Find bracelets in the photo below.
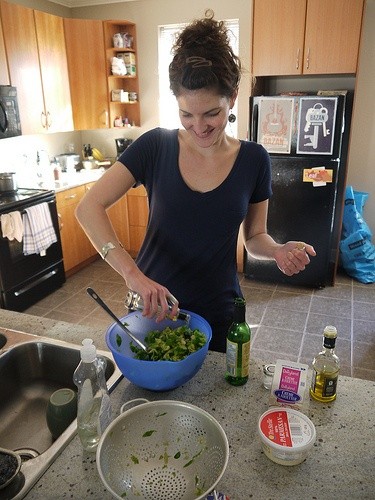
[100,241,124,260]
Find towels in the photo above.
[23,201,58,257]
[1,211,23,243]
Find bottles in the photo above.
[112,33,124,48]
[54,163,60,184]
[122,292,191,322]
[72,338,113,453]
[224,297,252,386]
[309,324,341,403]
[46,388,78,439]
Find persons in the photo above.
[74,19,316,353]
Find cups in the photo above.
[262,363,275,390]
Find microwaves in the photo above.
[0,86,22,140]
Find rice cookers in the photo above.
[54,153,81,174]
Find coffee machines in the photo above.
[115,138,132,160]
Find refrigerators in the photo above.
[243,92,354,290]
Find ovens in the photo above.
[0,200,66,312]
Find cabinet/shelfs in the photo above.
[251,0,365,77]
[0,0,143,135]
[57,178,151,280]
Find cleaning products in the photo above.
[73,338,113,454]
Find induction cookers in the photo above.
[0,188,56,216]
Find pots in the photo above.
[81,160,111,169]
[0,172,17,192]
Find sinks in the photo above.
[0,333,7,351]
[0,341,123,500]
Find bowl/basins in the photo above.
[104,308,212,392]
[258,407,316,467]
